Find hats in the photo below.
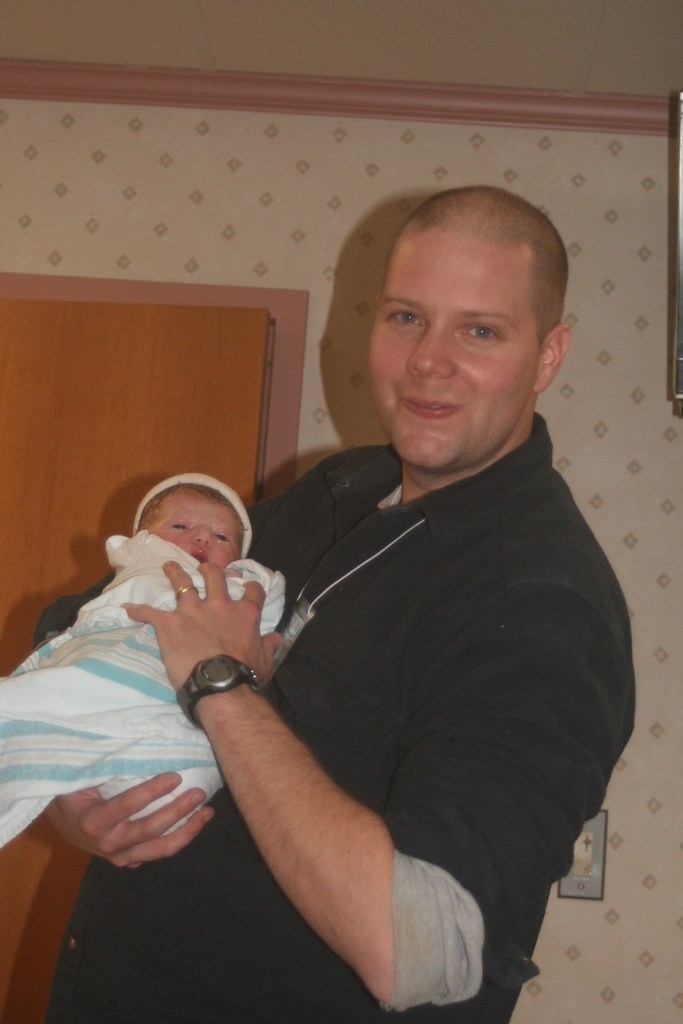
[132,472,252,559]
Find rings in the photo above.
[176,585,198,599]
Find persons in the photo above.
[0,473,285,850]
[34,185,636,1024]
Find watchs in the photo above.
[176,654,260,729]
